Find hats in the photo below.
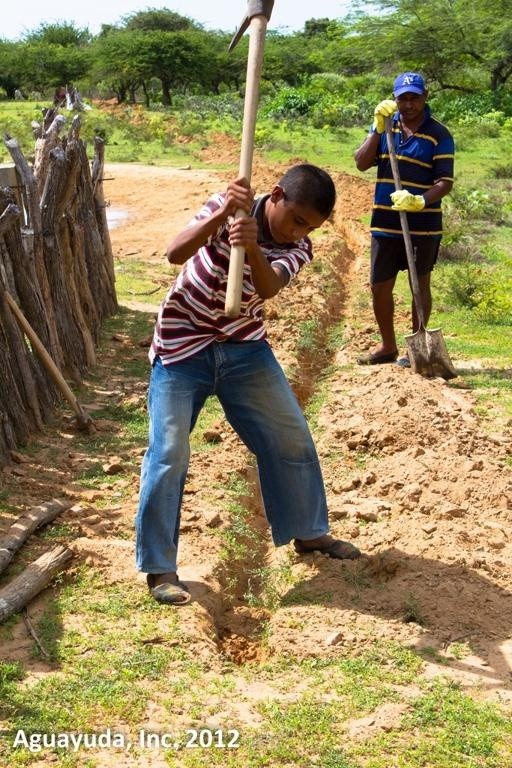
[393,72,425,97]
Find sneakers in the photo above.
[356,348,412,368]
[146,573,192,605]
[293,535,362,560]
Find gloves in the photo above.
[389,189,426,213]
[373,100,398,134]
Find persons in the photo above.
[136,164,361,606]
[354,72,455,368]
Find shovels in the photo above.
[384,114,458,379]
